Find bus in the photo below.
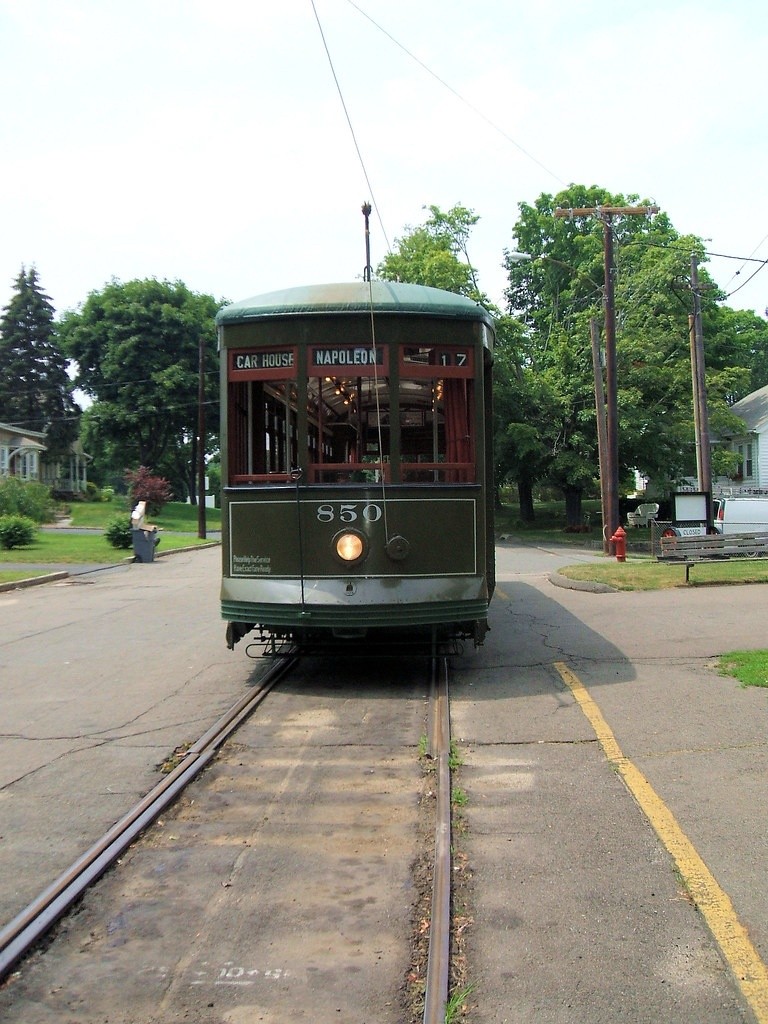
[215,201,497,665]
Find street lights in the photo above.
[508,251,619,558]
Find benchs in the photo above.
[656,531,768,583]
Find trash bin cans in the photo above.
[129,528,157,562]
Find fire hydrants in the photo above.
[609,525,628,564]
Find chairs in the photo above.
[627,503,660,528]
